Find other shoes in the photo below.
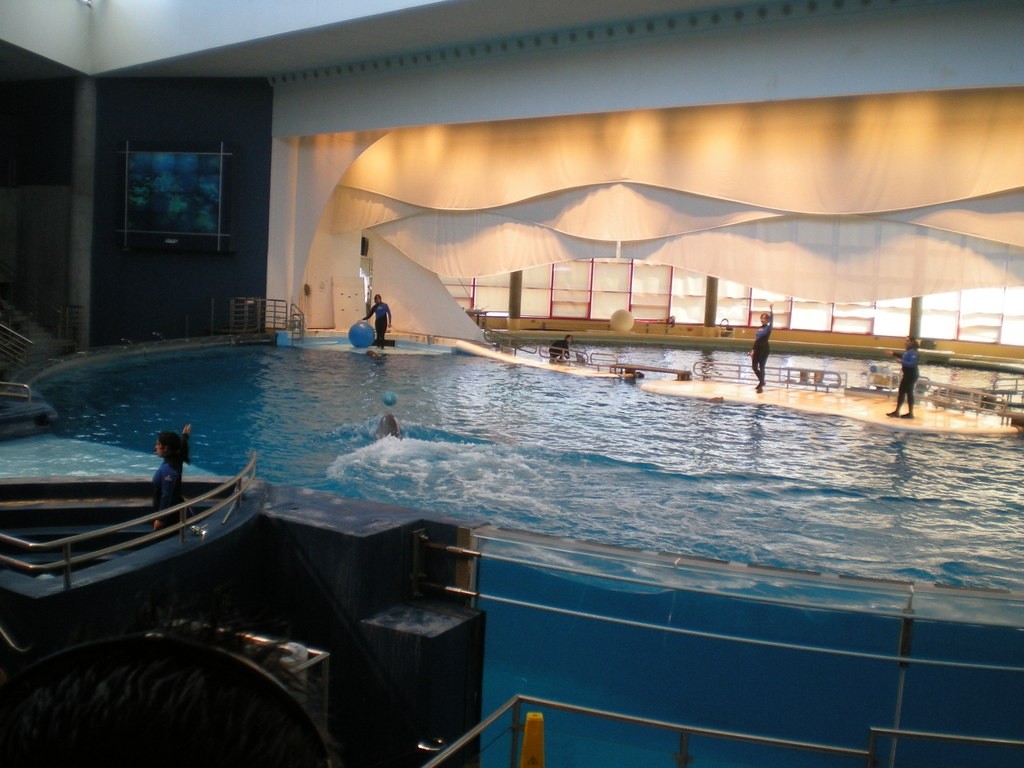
[901,413,913,418]
[887,412,899,417]
[756,383,765,389]
[757,388,762,393]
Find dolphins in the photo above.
[373,411,402,440]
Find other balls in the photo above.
[383,390,399,407]
[349,322,376,348]
[610,310,635,332]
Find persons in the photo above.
[153,424,192,538]
[358,294,391,349]
[549,335,573,363]
[883,336,920,418]
[750,303,774,393]
